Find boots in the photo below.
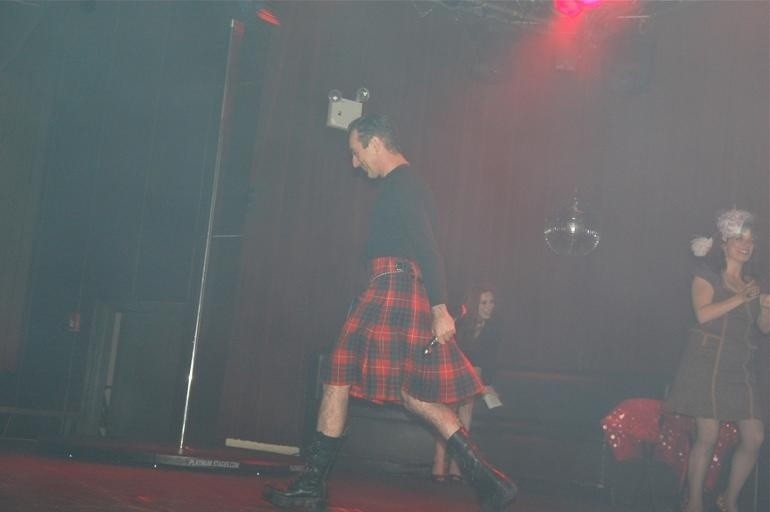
[446,425,518,511]
[262,430,344,512]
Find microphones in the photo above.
[423,303,469,356]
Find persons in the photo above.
[258,110,519,512]
[429,285,502,485]
[675,206,770,512]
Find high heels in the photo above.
[431,459,462,484]
[682,493,727,511]
